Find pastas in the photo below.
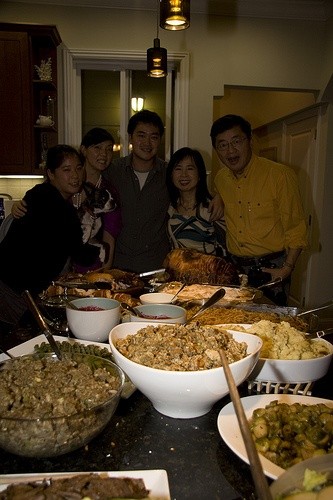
[182,305,310,333]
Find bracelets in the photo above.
[283,261,296,271]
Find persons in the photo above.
[0,109,308,332]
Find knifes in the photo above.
[25,290,63,361]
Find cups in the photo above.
[39,115,54,127]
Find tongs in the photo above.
[54,267,113,290]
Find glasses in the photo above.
[216,137,247,151]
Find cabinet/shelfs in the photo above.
[0,22,62,175]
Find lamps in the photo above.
[146,6,168,77]
[160,0,190,32]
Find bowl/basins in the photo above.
[140,293,178,305]
[215,324,333,383]
[0,352,125,460]
[130,304,187,324]
[109,322,264,418]
[269,454,333,500]
[66,298,121,342]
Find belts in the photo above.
[234,252,284,266]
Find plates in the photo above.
[114,279,145,293]
[155,284,321,334]
[0,470,170,500]
[0,334,136,397]
[217,394,333,480]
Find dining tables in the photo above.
[0,271,333,500]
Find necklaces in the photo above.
[179,204,195,211]
